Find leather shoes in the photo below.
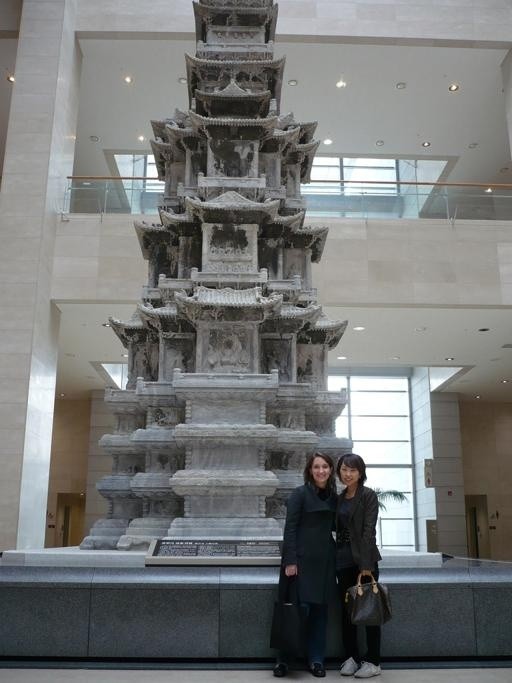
[273,660,290,677]
[308,663,326,677]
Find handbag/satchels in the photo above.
[269,598,310,659]
[344,571,392,626]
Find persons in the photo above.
[335,454,382,678]
[273,451,339,677]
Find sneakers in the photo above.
[354,661,381,678]
[338,658,359,675]
